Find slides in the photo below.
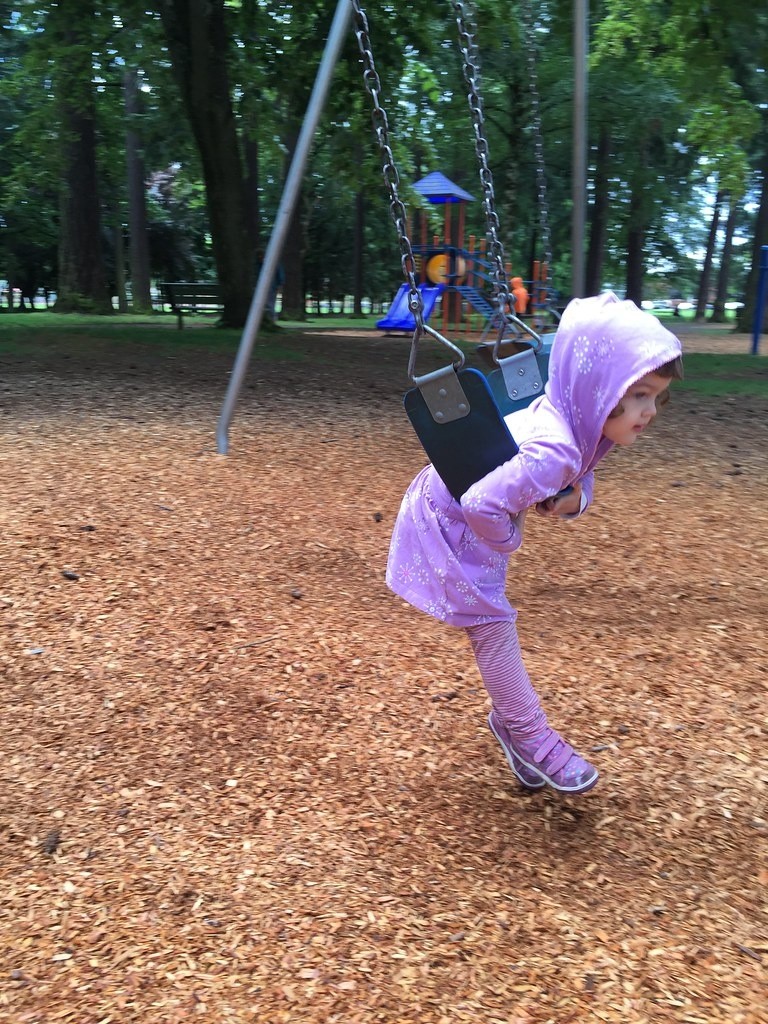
[377,281,444,334]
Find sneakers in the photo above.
[487,710,546,789]
[510,728,599,794]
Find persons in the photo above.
[508,275,529,313]
[385,290,685,792]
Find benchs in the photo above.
[157,283,225,329]
[515,314,545,336]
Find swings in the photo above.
[351,0,573,506]
